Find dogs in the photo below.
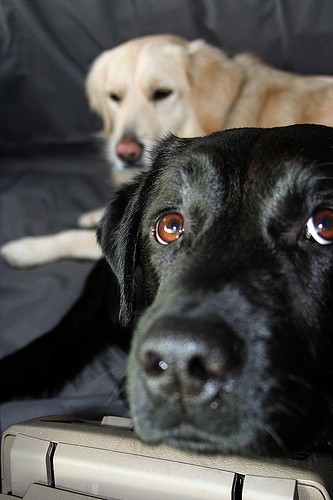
[0,30,332,269]
[0,122,331,461]
